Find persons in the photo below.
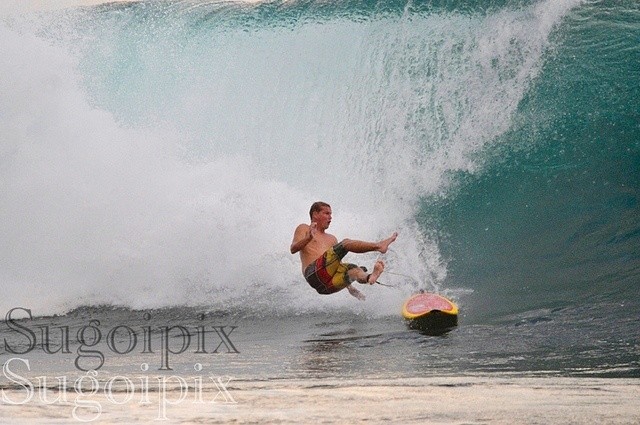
[290,202,399,301]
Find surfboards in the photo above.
[403,294,457,320]
